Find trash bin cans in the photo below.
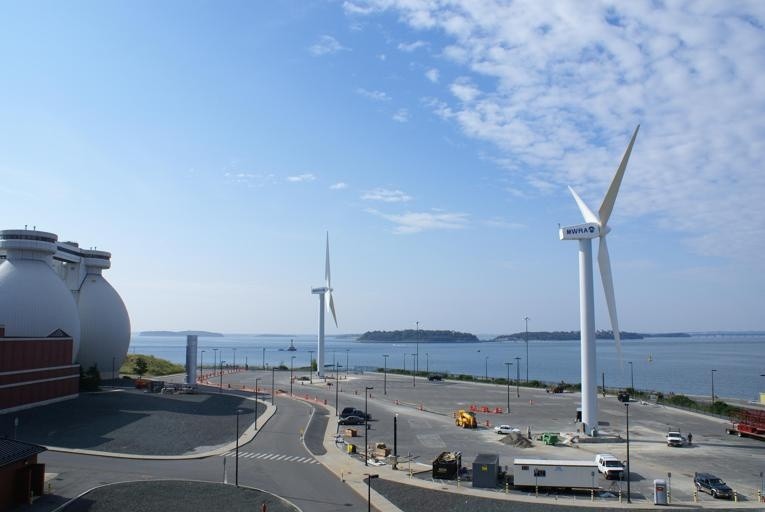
[654,479,667,505]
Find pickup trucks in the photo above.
[596,453,625,480]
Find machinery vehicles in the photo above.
[455,409,478,428]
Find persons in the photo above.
[688,432,692,441]
[528,426,532,439]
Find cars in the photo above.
[494,425,522,435]
[337,407,372,427]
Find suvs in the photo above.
[666,431,684,447]
[693,470,732,500]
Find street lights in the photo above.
[363,387,375,466]
[504,361,511,413]
[486,356,489,382]
[711,369,717,405]
[627,362,634,394]
[514,357,521,397]
[384,320,431,401]
[624,403,632,504]
[368,474,379,512]
[523,315,531,384]
[334,361,343,416]
[198,346,315,491]
[345,349,351,375]
